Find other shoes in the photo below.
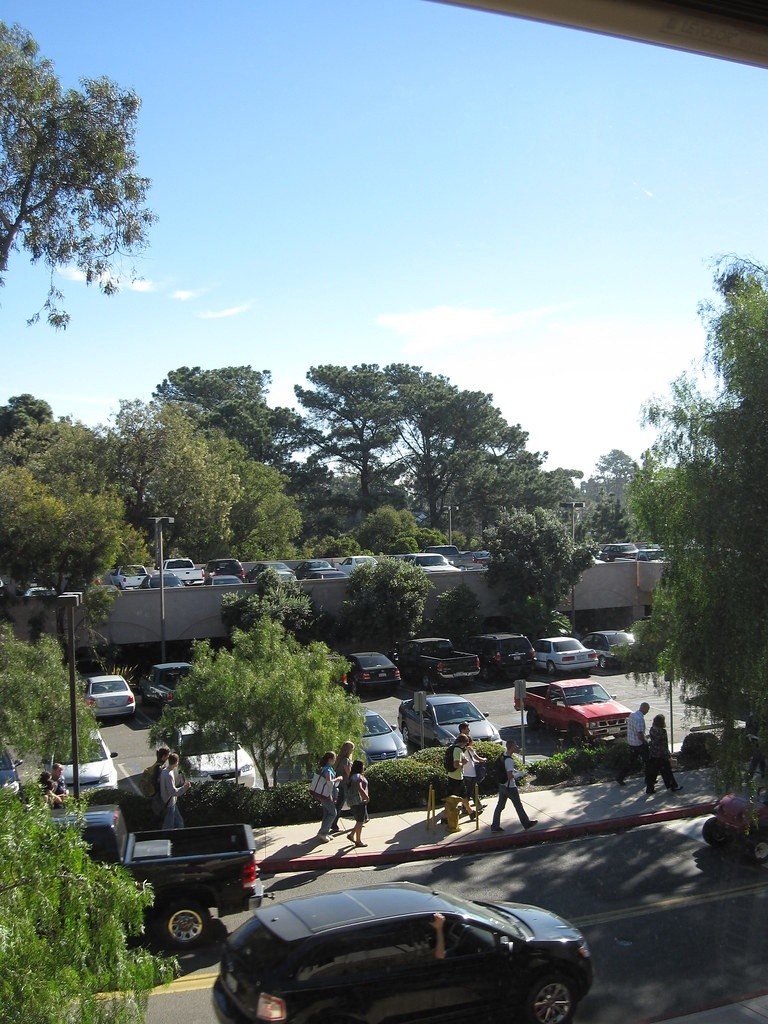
[348,835,355,843]
[333,827,342,832]
[469,810,483,821]
[461,805,469,814]
[645,778,659,784]
[440,817,448,824]
[491,825,504,832]
[646,789,656,794]
[318,832,333,842]
[525,821,538,831]
[615,777,625,785]
[478,804,487,810]
[356,841,367,847]
[672,786,683,792]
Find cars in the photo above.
[397,694,504,749]
[20,541,665,601]
[358,710,410,768]
[346,650,402,696]
[80,673,136,720]
[40,724,119,798]
[210,882,595,1024]
[579,630,637,672]
[163,720,255,790]
[0,742,23,795]
[528,636,600,674]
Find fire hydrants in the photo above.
[443,794,464,833]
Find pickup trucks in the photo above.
[392,638,481,691]
[46,801,265,951]
[140,662,199,710]
[513,679,633,750]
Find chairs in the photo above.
[94,686,107,694]
[110,683,128,691]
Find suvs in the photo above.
[464,632,538,684]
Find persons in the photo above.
[346,760,369,848]
[316,751,343,843]
[646,713,683,794]
[615,702,660,786]
[439,722,488,824]
[150,748,170,829]
[490,739,538,832]
[330,741,369,833]
[36,765,71,809]
[160,754,191,828]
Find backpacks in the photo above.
[494,752,513,784]
[140,763,162,798]
[444,745,461,772]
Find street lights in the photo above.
[57,592,82,802]
[442,500,586,633]
[144,516,177,658]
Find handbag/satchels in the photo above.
[341,800,351,811]
[151,796,168,818]
[474,761,488,784]
[310,769,334,800]
[347,775,362,806]
[668,756,677,768]
